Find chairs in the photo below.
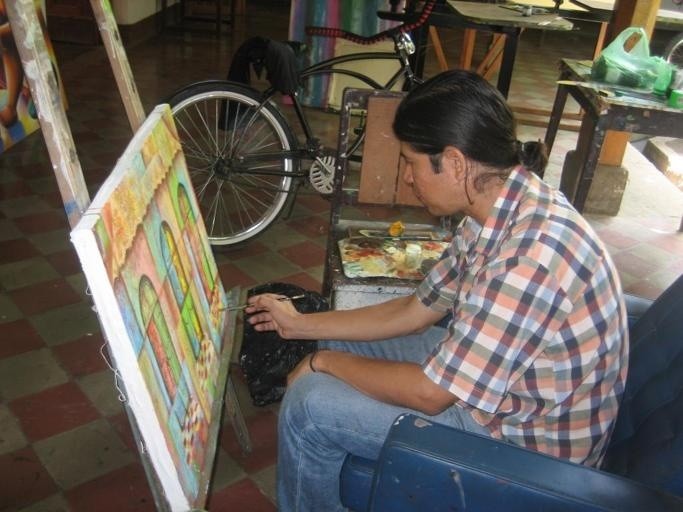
[322,87,446,298]
[338,293,683,512]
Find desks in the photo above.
[427,0,683,132]
[537,58,683,231]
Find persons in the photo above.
[238,65,628,511]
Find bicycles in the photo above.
[160,0,438,250]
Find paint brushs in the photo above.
[369,237,430,240]
[220,294,304,312]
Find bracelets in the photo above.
[308,346,331,373]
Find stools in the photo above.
[161,0,235,40]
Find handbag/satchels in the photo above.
[239,282,329,404]
